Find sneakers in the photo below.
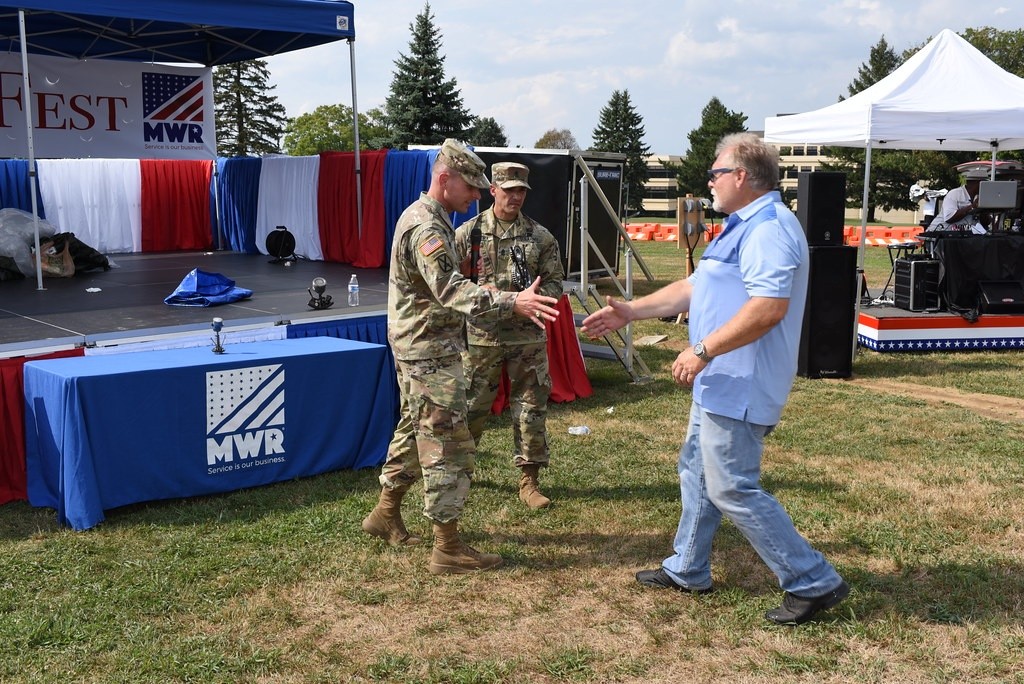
[765,581,850,627]
[636,569,714,596]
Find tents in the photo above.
[0,0,362,291]
[763,28,1024,363]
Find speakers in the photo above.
[971,280,1024,314]
[796,172,859,380]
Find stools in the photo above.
[880,242,917,294]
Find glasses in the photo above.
[707,168,736,182]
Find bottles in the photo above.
[347,274,359,306]
[568,426,591,435]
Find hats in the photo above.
[491,162,532,190]
[966,169,988,180]
[436,138,492,189]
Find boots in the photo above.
[361,486,421,551]
[519,465,552,510]
[430,519,503,578]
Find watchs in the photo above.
[693,341,714,362]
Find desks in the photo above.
[23,336,396,531]
[914,235,1024,310]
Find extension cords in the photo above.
[872,299,894,305]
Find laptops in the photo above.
[970,181,1017,213]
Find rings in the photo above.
[536,311,541,317]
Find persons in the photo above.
[451,162,565,510]
[925,170,989,254]
[361,138,560,575]
[579,132,850,625]
